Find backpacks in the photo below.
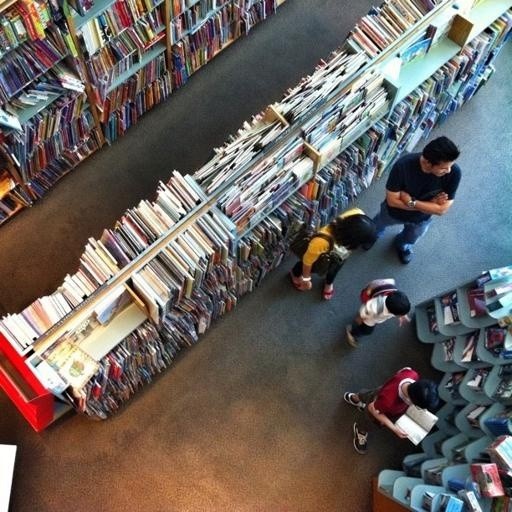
[360,278,398,313]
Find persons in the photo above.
[344,366,440,455]
[374,134,462,265]
[287,207,379,299]
[196,1,512,237]
[345,278,412,349]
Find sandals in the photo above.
[288,270,305,292]
[322,283,333,300]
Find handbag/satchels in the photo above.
[290,234,335,276]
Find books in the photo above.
[0,174,263,424]
[372,267,512,511]
[0,0,286,226]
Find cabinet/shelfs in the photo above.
[374,264,512,512]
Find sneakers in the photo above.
[344,392,366,412]
[398,244,411,264]
[353,421,369,454]
